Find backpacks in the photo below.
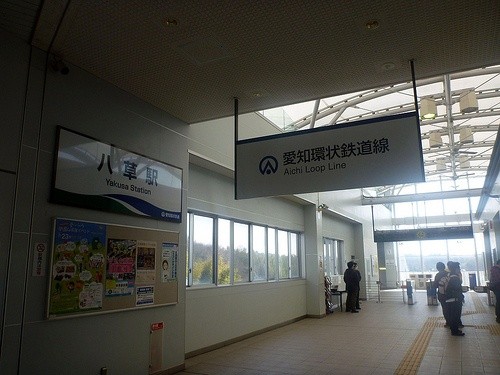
[439,273,456,294]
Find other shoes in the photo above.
[356,307,361,309]
[458,324,464,328]
[451,330,465,336]
[444,324,450,329]
[352,310,359,313]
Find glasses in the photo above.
[353,265,357,267]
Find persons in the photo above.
[432,262,466,337]
[489,260,500,323]
[344,262,361,313]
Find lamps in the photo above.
[419,95,438,120]
[429,130,443,149]
[436,158,448,172]
[461,127,474,145]
[460,158,470,170]
[459,89,480,116]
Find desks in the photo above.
[333,289,347,311]
